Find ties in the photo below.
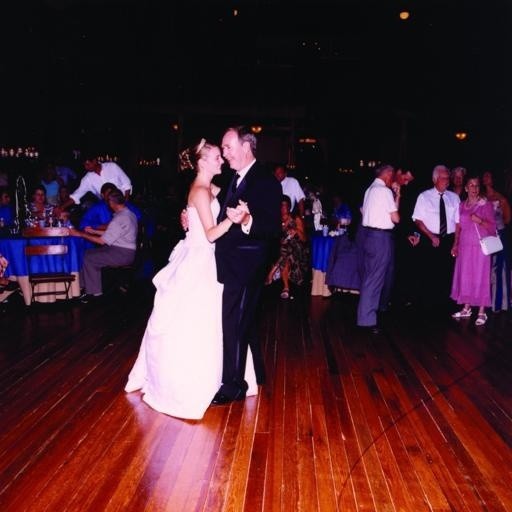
[440,194,447,238]
[232,175,240,192]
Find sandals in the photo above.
[475,313,488,326]
[452,308,472,317]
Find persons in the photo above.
[0,150,144,312]
[264,162,512,335]
[120,133,260,421]
[181,121,284,407]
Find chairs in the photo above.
[22,227,76,326]
[101,226,146,304]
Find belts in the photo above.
[363,227,392,232]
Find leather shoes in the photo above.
[212,385,245,404]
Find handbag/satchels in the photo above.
[480,236,504,255]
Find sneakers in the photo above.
[279,288,290,300]
[80,294,103,304]
